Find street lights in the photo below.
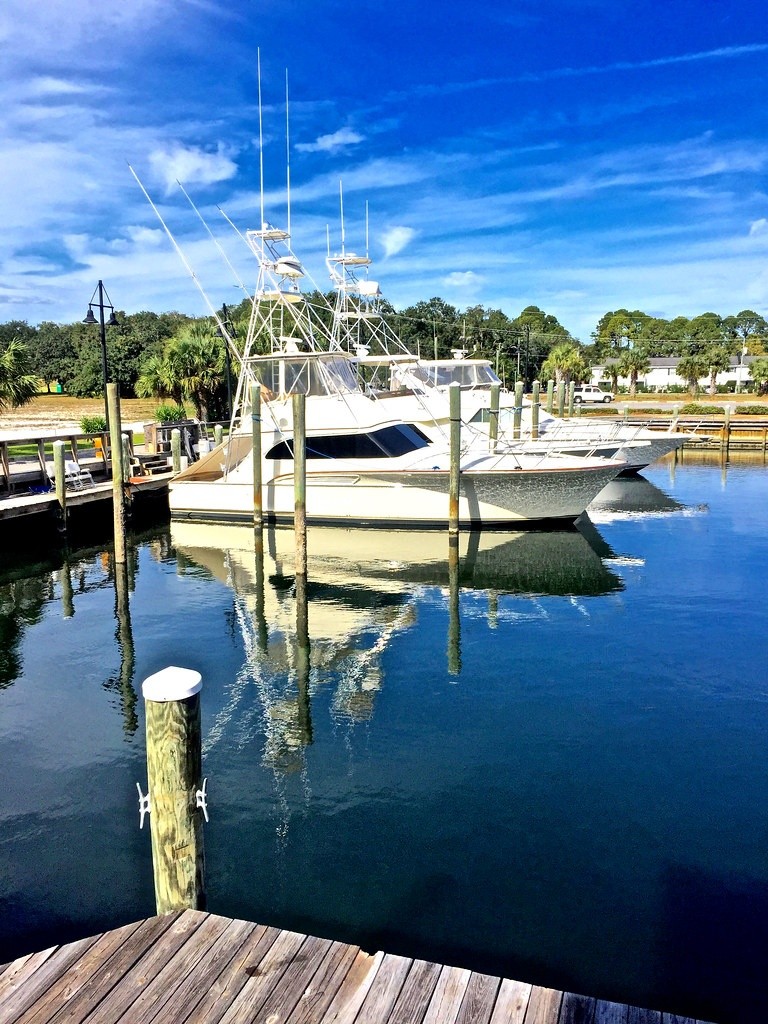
[81,278,120,441]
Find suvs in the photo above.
[569,382,614,403]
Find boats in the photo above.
[125,38,622,523]
[319,174,698,483]
[168,469,710,784]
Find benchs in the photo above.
[44,460,97,493]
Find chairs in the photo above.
[129,456,143,477]
